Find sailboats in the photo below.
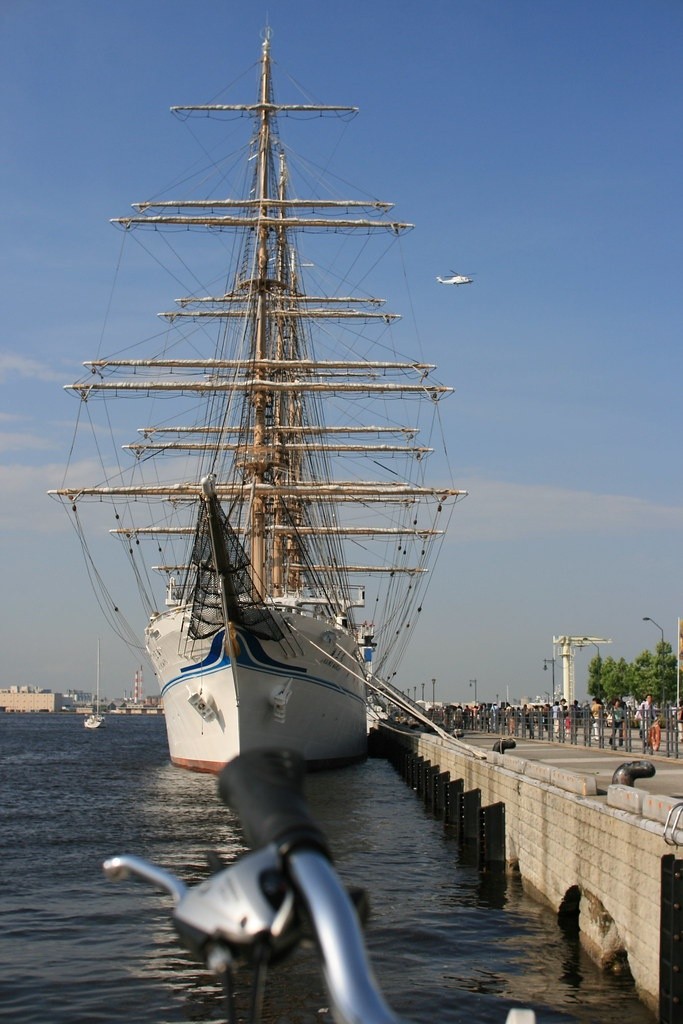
[44,8,473,777]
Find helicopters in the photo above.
[436,269,479,286]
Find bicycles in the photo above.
[99,745,541,1024]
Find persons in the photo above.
[434,695,682,753]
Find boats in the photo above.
[84,713,105,729]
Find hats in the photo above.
[582,703,589,708]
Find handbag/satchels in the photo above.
[607,715,612,726]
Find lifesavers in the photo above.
[649,723,661,751]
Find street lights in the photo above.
[641,615,665,709]
[400,678,436,714]
[542,657,556,706]
[582,637,601,701]
[469,677,477,706]
